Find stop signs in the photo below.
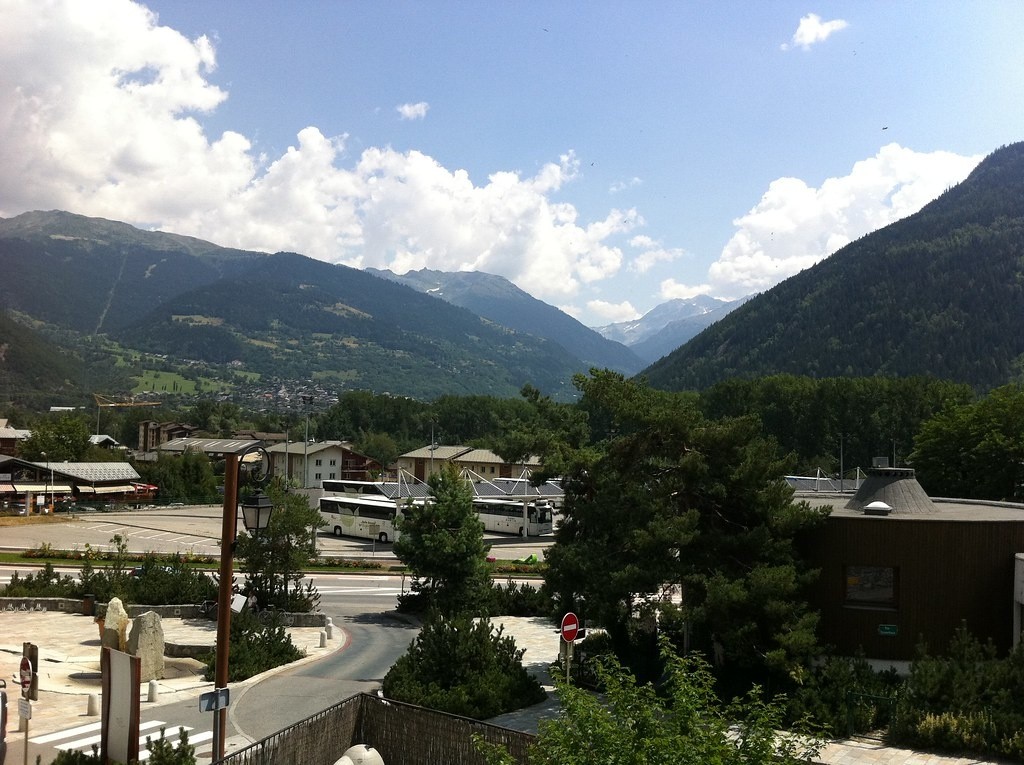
[561,612,578,643]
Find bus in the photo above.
[320,480,400,499]
[472,499,554,536]
[316,496,425,545]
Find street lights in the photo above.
[150,433,274,765]
[40,452,50,508]
[301,395,314,487]
